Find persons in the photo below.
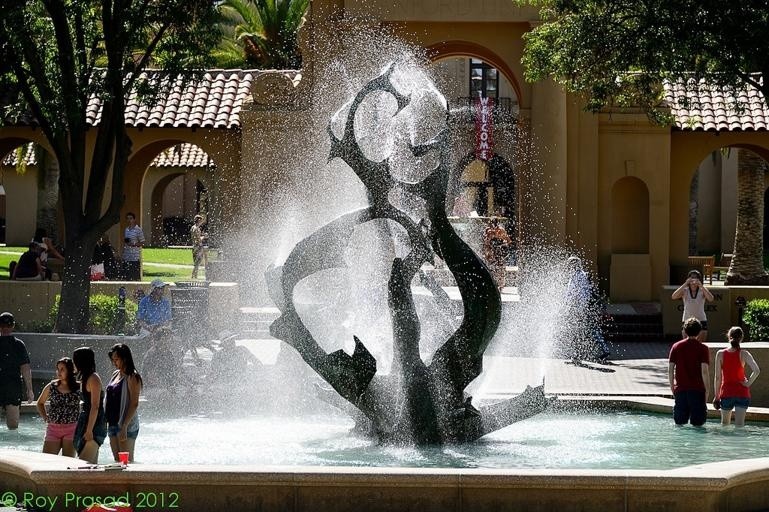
[483,217,513,288]
[564,257,611,364]
[669,317,710,427]
[713,326,761,425]
[671,270,714,344]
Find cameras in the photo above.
[690,279,699,284]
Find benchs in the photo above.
[704,251,733,281]
[687,253,716,285]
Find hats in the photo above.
[0,311,16,323]
[150,278,170,290]
[217,329,241,347]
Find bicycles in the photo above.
[172,310,222,388]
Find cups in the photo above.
[118,451,130,466]
